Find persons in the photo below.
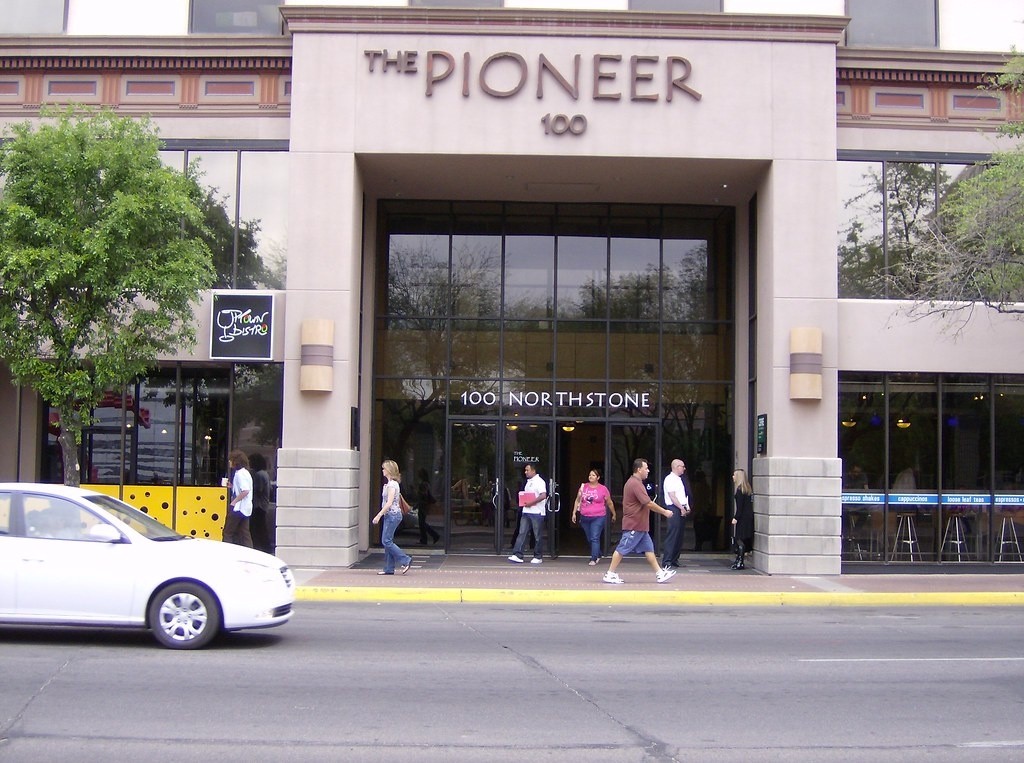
[729,469,754,570]
[955,487,976,536]
[508,462,548,564]
[222,451,254,548]
[602,459,677,584]
[248,453,272,553]
[661,459,690,569]
[690,470,713,553]
[412,467,441,544]
[842,461,923,553]
[572,469,617,565]
[371,460,413,575]
[481,479,537,550]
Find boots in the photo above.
[728,544,745,570]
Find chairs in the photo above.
[27,508,68,539]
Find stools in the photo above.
[840,508,1023,564]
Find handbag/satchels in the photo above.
[398,494,410,515]
[428,489,437,504]
[577,484,585,511]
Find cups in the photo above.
[221,478,228,487]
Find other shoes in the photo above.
[432,534,441,544]
[403,559,412,574]
[589,556,602,566]
[416,541,427,546]
[672,561,686,567]
[378,570,394,575]
[664,564,671,568]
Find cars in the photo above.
[0,476,296,648]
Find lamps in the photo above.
[842,415,856,427]
[896,406,910,428]
[300,318,335,391]
[789,326,823,400]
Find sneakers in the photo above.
[656,565,676,583]
[507,555,523,563]
[602,571,624,584]
[531,558,542,563]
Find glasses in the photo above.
[679,465,685,469]
